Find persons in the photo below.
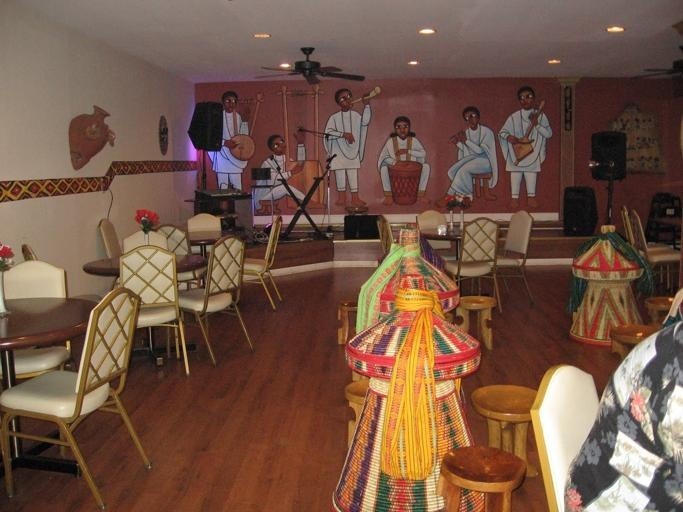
[377,116,431,207]
[254,135,322,210]
[208,90,248,191]
[563,317,683,511]
[320,88,372,210]
[497,86,552,209]
[435,104,497,208]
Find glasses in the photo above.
[395,124,410,129]
[338,94,352,103]
[518,94,534,100]
[463,113,477,122]
[223,99,239,103]
[271,139,284,151]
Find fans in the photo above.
[633,21,682,80]
[254,46,365,85]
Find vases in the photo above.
[144,229,150,248]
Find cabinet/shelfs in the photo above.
[197,188,251,244]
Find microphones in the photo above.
[326,154,337,162]
[271,154,276,160]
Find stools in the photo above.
[457,295,496,355]
[646,296,677,322]
[609,322,657,363]
[441,442,526,511]
[335,300,359,347]
[471,382,542,479]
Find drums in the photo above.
[230,134,255,160]
[389,160,422,206]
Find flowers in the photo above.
[442,195,471,209]
[134,208,159,232]
[0,242,14,272]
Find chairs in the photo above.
[2,287,152,507]
[376,209,538,312]
[20,245,37,261]
[120,244,192,376]
[178,235,255,366]
[122,230,169,255]
[529,362,604,511]
[97,218,121,258]
[620,205,681,297]
[0,260,78,455]
[236,214,283,309]
[155,225,189,256]
[187,212,223,232]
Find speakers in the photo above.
[592,131,626,180]
[344,215,382,240]
[563,187,598,236]
[187,102,223,151]
